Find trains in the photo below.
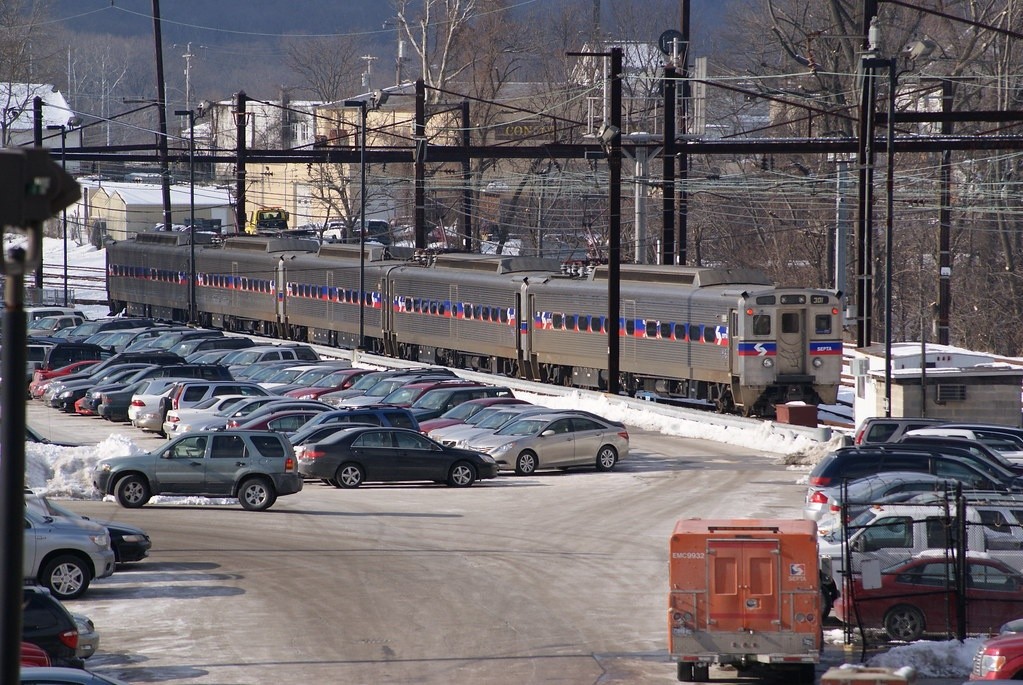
[101,221,840,420]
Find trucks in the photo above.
[667,514,842,685]
[246,205,316,238]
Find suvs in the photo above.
[409,382,516,421]
[68,316,324,364]
[20,584,79,661]
[326,365,492,409]
[809,443,1023,493]
[90,429,305,513]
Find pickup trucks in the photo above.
[320,222,361,244]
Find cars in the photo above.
[855,488,1023,551]
[818,663,916,685]
[418,396,536,437]
[238,355,381,401]
[964,630,1023,681]
[898,435,1023,475]
[23,479,154,566]
[429,403,549,445]
[25,338,424,446]
[485,182,512,197]
[71,611,99,659]
[23,504,115,600]
[896,425,1023,468]
[18,663,133,685]
[802,471,979,520]
[840,548,1023,642]
[25,493,53,515]
[816,504,987,594]
[288,427,500,489]
[470,408,629,476]
[394,216,510,246]
[150,223,223,245]
[999,618,1023,637]
[853,416,958,445]
[353,219,393,245]
[17,642,52,668]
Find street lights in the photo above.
[860,52,897,416]
[564,45,624,394]
[342,96,373,352]
[46,123,68,307]
[174,105,196,326]
